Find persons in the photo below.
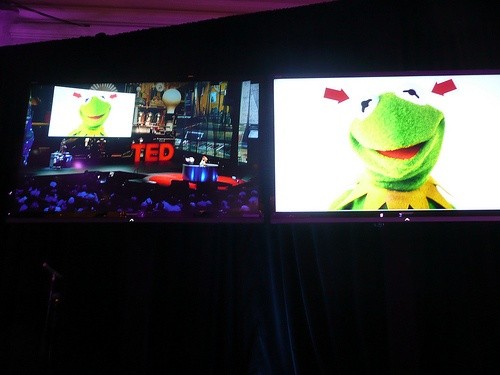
[7,138,273,220]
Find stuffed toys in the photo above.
[70,91,118,137]
[327,81,460,211]
